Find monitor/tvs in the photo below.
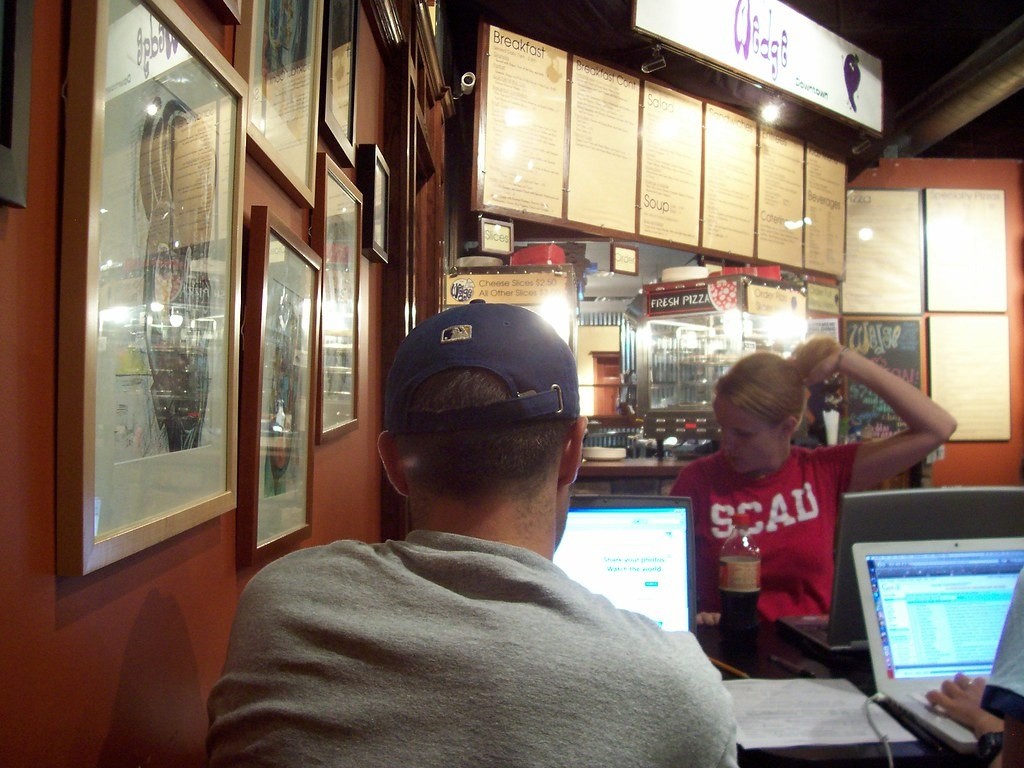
[551,492,695,635]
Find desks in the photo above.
[701,622,986,768]
[577,455,695,494]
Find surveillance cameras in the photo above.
[461,72,476,94]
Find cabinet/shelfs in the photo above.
[634,274,809,422]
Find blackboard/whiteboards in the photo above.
[841,316,928,446]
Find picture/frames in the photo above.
[318,0,360,169]
[0,0,34,211]
[54,0,249,581]
[310,151,363,445]
[203,0,242,28]
[839,187,927,317]
[609,243,640,276]
[231,0,323,211]
[477,217,515,256]
[358,141,393,267]
[237,202,323,577]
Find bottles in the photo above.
[718,514,762,672]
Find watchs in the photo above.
[976,730,1003,768]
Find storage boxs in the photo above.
[509,243,566,265]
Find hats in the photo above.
[383,299,581,437]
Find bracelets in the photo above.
[835,344,850,371]
[964,677,975,689]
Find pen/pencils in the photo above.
[709,657,750,679]
[769,654,816,678]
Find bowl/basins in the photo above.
[582,447,626,461]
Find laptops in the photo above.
[773,487,1024,754]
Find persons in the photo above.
[925,568,1024,768]
[205,299,739,768]
[666,332,958,626]
[620,370,636,415]
[790,384,831,446]
